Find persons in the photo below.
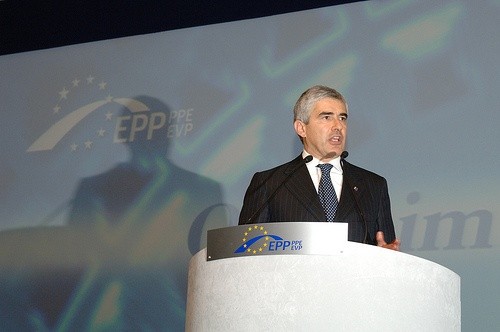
[237,86,399,253]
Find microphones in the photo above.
[246,153,313,223]
[339,148,372,243]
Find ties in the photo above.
[316,162,341,222]
